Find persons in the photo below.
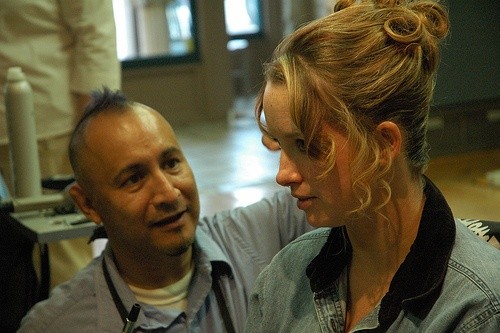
[1,0,125,332]
[17,83,317,333]
[245,0,499,333]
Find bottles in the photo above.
[5,66,46,198]
